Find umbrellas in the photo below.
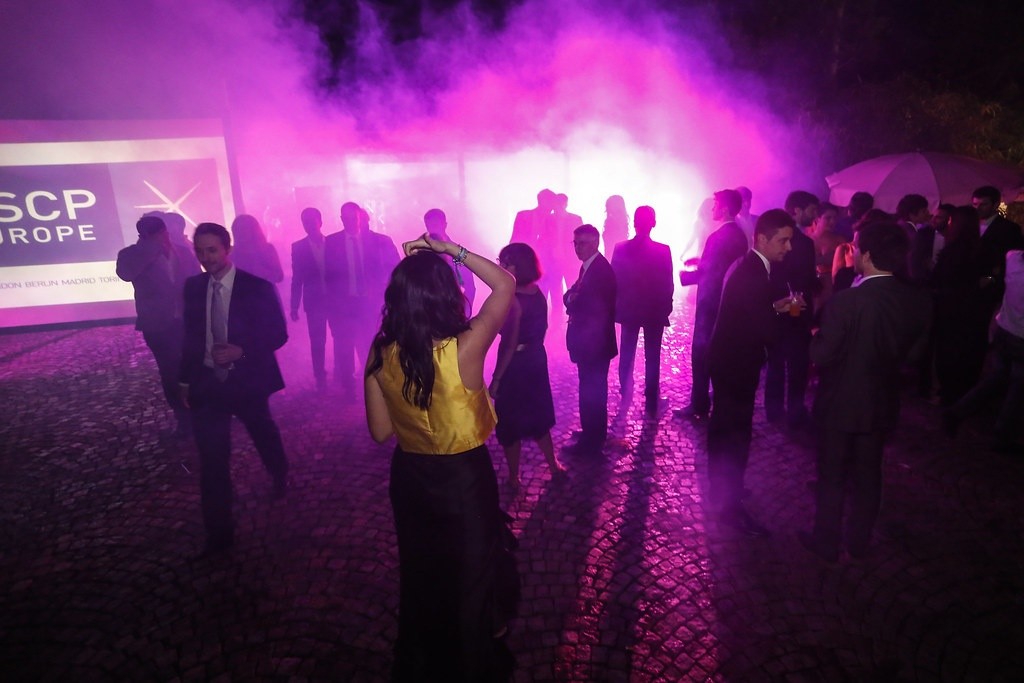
[826,148,1023,216]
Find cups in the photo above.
[789,292,803,315]
[210,344,231,369]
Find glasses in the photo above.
[496,258,516,269]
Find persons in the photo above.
[181,222,291,565]
[359,209,401,281]
[290,208,341,394]
[941,248,1024,455]
[484,241,568,490]
[562,225,618,456]
[781,179,1002,407]
[116,210,204,440]
[424,209,477,325]
[364,232,519,683]
[609,206,675,417]
[702,187,761,248]
[324,201,387,404]
[671,190,749,422]
[510,189,564,301]
[703,206,807,539]
[226,214,284,290]
[797,217,933,565]
[602,195,628,266]
[970,187,1023,281]
[550,194,583,334]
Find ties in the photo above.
[577,266,584,288]
[210,281,229,383]
[352,238,366,294]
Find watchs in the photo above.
[238,348,248,365]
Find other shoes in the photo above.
[507,479,523,494]
[561,441,605,455]
[272,473,291,498]
[806,480,845,495]
[571,431,583,440]
[175,420,193,437]
[767,407,785,421]
[551,469,568,481]
[799,533,839,564]
[845,531,872,559]
[644,398,669,417]
[672,403,711,418]
[205,528,234,557]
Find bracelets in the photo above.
[452,244,471,287]
